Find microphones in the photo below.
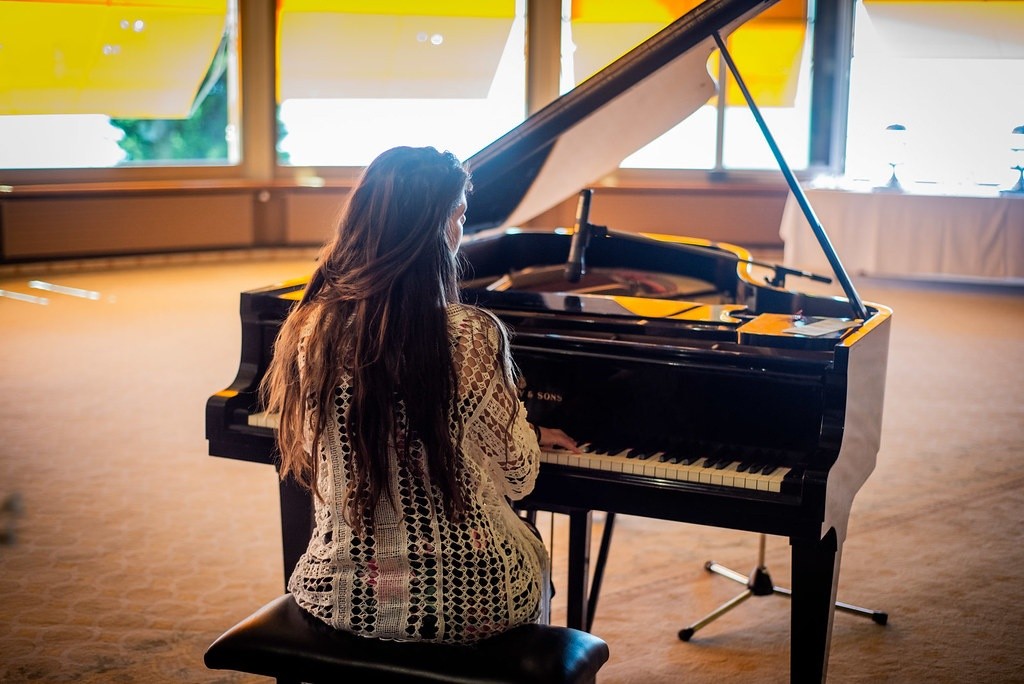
[564,189,594,285]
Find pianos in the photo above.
[203,1,892,684]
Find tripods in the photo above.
[581,223,890,640]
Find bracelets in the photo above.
[533,424,541,443]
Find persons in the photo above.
[262,147,583,643]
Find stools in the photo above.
[203,592,609,684]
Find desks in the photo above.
[776,173,1024,287]
